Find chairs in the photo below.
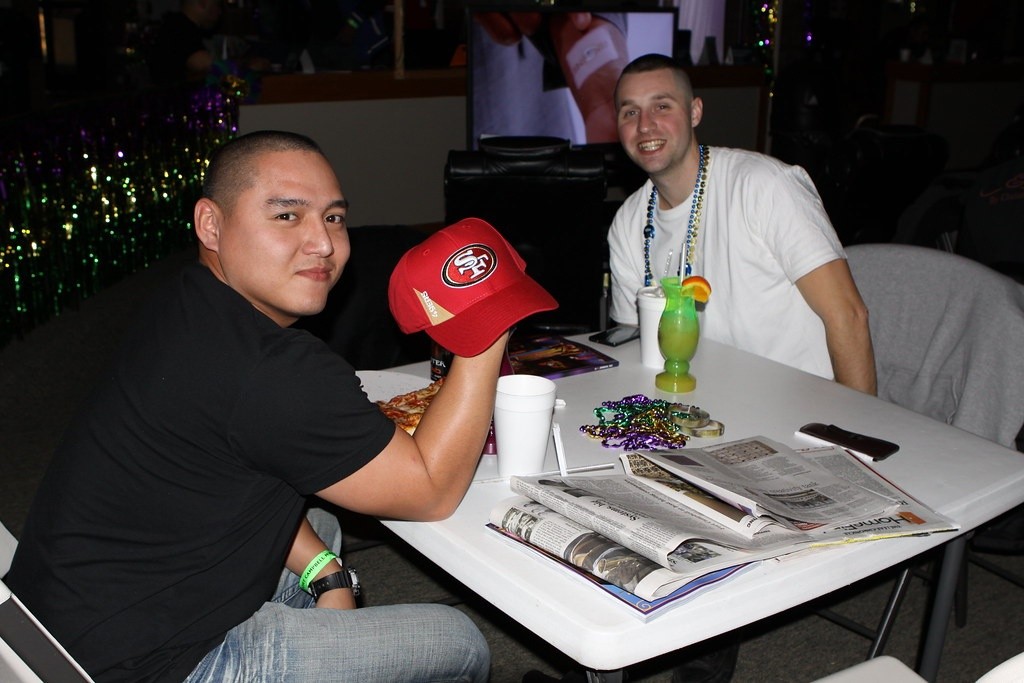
[840,243,1024,682]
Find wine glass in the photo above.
[655,276,700,393]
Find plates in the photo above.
[355,371,440,437]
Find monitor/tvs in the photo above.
[465,4,681,165]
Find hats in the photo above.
[388,217,559,359]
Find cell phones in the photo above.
[800,423,900,460]
[589,326,639,347]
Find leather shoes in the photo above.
[672,627,742,683]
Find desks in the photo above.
[335,318,1024,683]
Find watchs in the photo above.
[311,567,362,601]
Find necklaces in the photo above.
[644,145,710,286]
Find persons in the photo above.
[23,131,511,683]
[607,53,878,398]
[474,13,628,146]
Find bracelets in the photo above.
[299,550,343,596]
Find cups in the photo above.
[431,340,454,381]
[636,287,666,370]
[493,374,556,484]
